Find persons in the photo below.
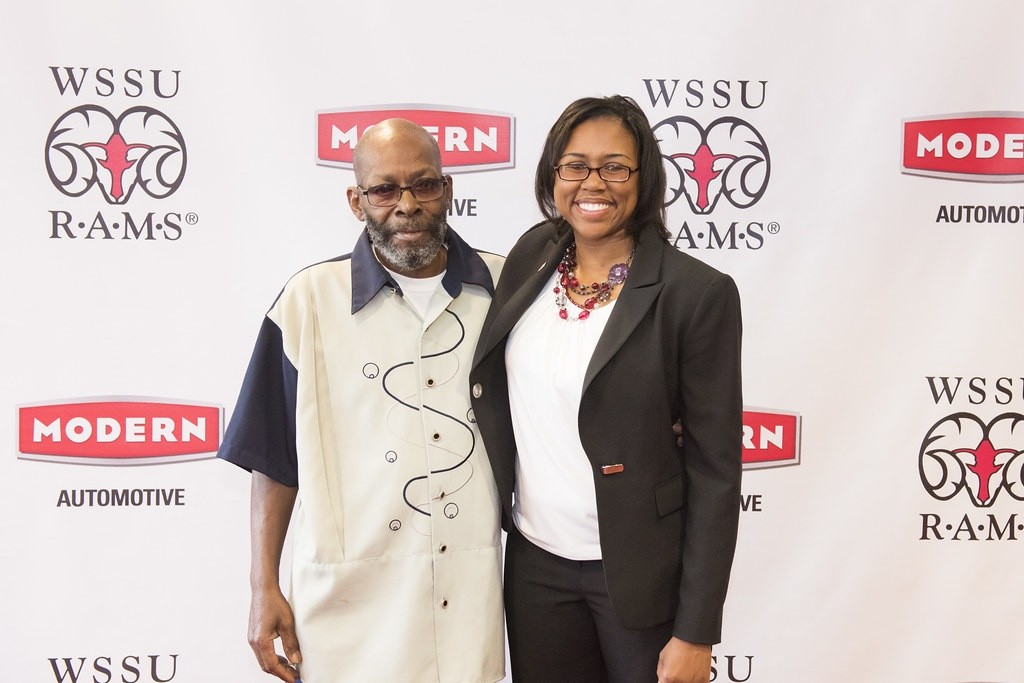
[216,118,682,683]
[468,96,741,683]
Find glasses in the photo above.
[551,160,641,182]
[357,175,447,207]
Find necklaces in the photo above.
[553,242,635,321]
